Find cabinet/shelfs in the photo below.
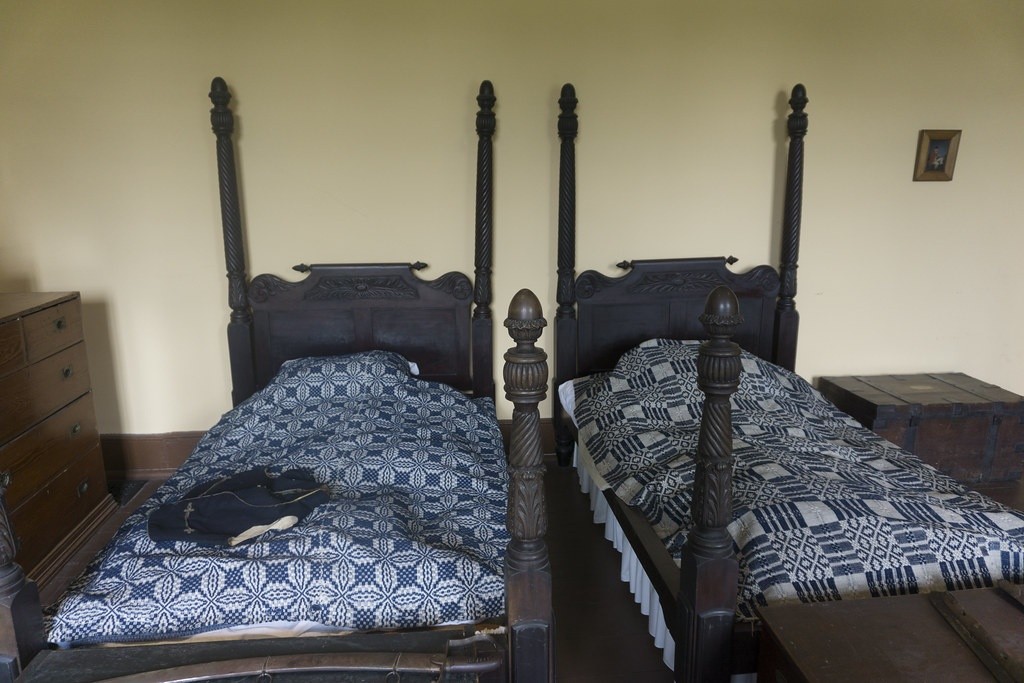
[0,290,118,595]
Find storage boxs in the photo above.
[818,371,1024,485]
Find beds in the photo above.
[0,73,556,683]
[557,84,1024,683]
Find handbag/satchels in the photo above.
[148,465,329,547]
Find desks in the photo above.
[755,581,1024,683]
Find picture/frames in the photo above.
[913,129,962,181]
